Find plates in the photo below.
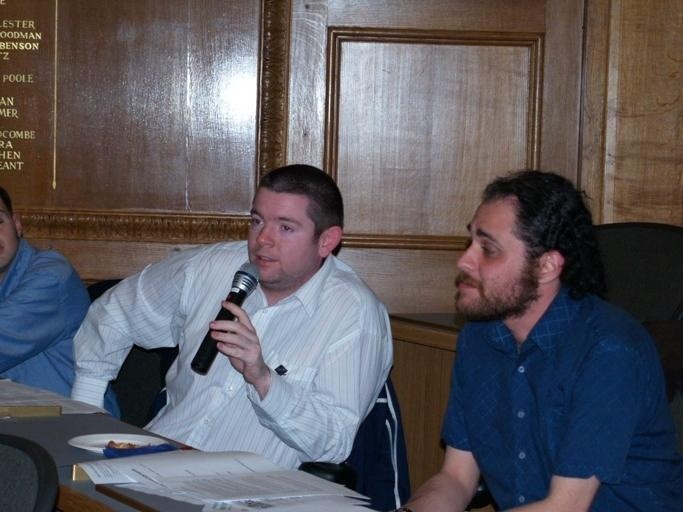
[68,433,171,454]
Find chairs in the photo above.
[461,223,683,510]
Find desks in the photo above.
[2,375,381,512]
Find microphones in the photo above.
[191,262,260,375]
